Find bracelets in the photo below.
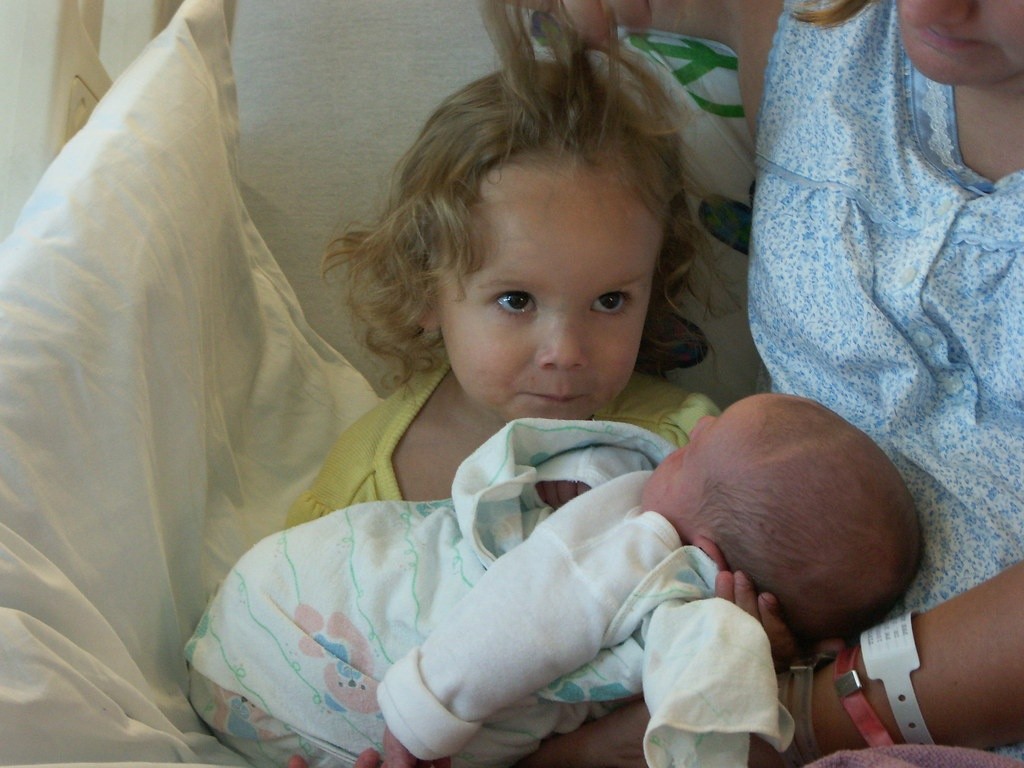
[859,608,937,746]
[834,644,896,750]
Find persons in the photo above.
[279,1,798,768]
[517,2,1023,768]
[184,392,928,768]
